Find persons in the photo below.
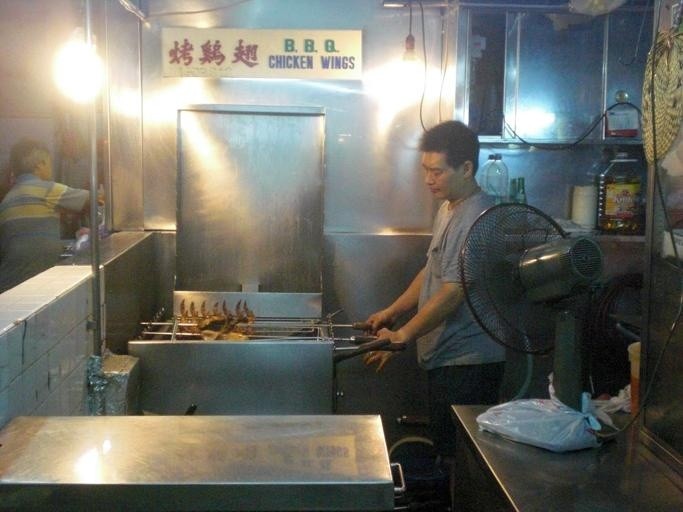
[0,137,104,294]
[364,120,507,487]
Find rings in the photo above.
[367,350,372,357]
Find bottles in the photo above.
[479,155,494,193]
[486,154,509,205]
[515,176,529,205]
[510,179,517,204]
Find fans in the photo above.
[455,200,611,450]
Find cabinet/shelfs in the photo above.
[452,0,656,144]
